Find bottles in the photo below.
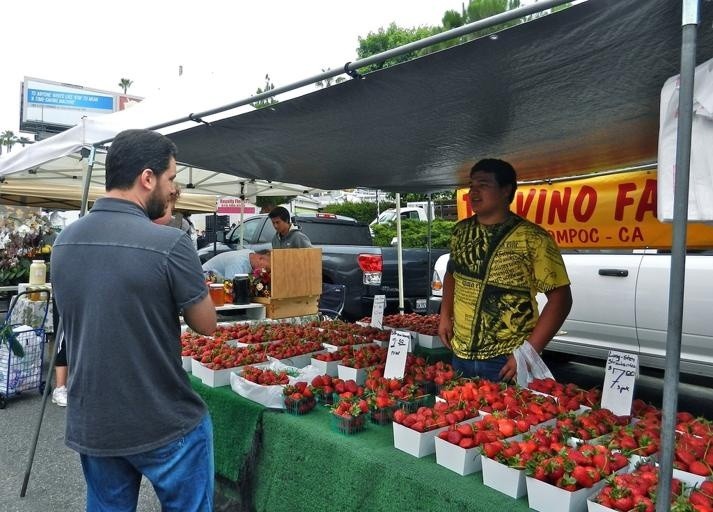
[26,284,53,300]
[29,260,46,284]
[209,283,224,306]
[231,272,250,306]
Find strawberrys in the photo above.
[181,320,392,370]
[283,354,455,434]
[360,313,440,336]
[393,377,713,512]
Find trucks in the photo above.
[365,197,459,238]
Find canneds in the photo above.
[27,260,53,301]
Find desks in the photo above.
[183,347,713,512]
[6,295,55,346]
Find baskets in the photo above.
[311,373,370,403]
[278,392,316,416]
[327,404,367,434]
[369,360,459,426]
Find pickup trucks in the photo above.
[191,211,451,336]
[422,196,713,405]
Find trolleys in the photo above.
[0,286,56,407]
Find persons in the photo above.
[151,183,191,236]
[51,297,67,406]
[201,249,271,282]
[439,159,573,386]
[269,207,313,248]
[49,130,217,512]
[196,230,209,250]
[38,226,58,249]
[24,224,40,248]
